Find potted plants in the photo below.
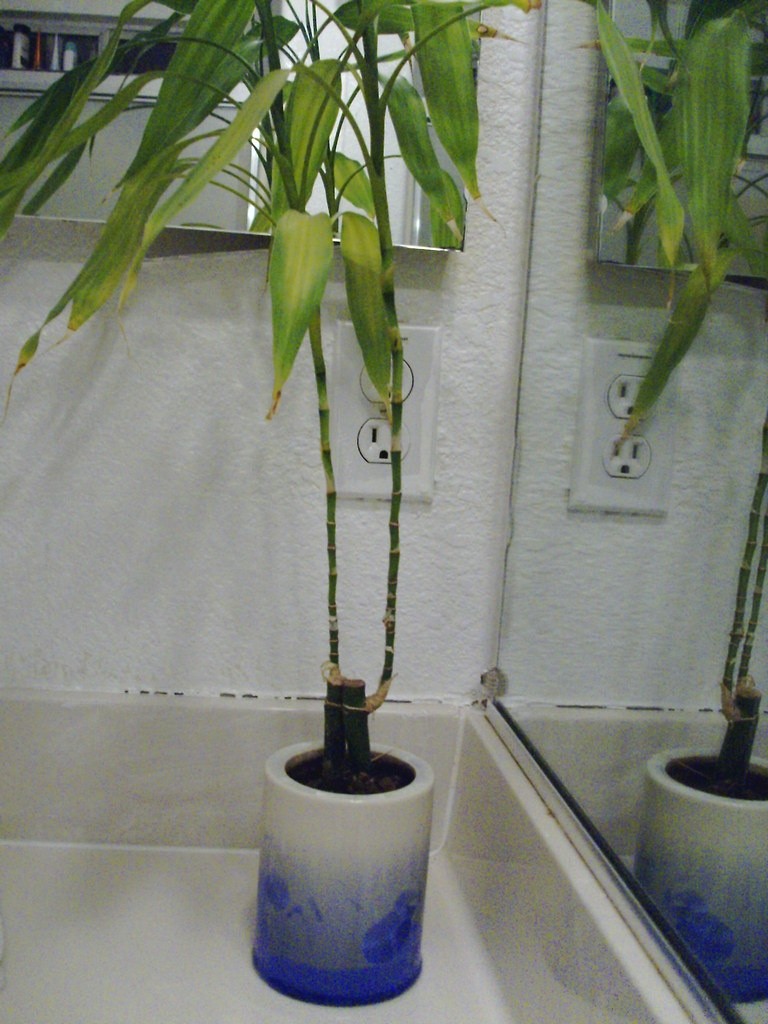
[585,0,768,1002]
[0,2,546,1009]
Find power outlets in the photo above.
[570,337,680,518]
[323,321,438,504]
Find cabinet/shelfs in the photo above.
[0,0,483,253]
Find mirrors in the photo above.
[487,2,768,1024]
